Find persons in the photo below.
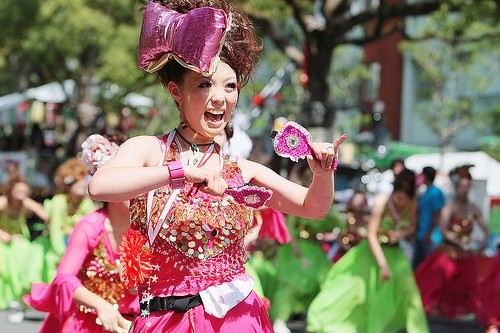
[0,0,499,333]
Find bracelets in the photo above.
[167,160,186,189]
